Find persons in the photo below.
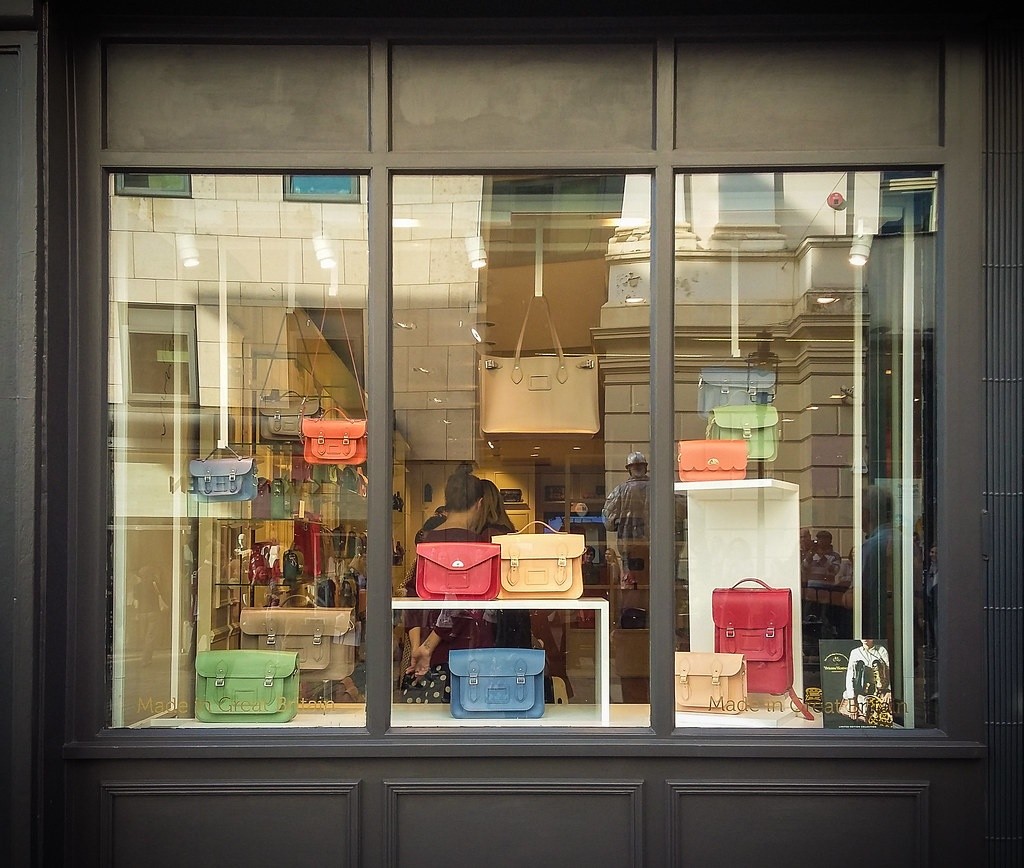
[799,487,937,641]
[846,640,889,721]
[602,451,651,628]
[403,469,576,705]
[576,546,620,628]
[126,534,253,664]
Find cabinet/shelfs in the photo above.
[563,453,689,680]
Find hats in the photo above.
[816,531,832,537]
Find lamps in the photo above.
[465,235,488,269]
[177,233,200,267]
[849,229,874,266]
[313,231,336,268]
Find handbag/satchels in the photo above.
[478,354,601,441]
[448,648,548,720]
[302,409,366,466]
[545,485,564,501]
[239,594,356,681]
[248,464,405,703]
[188,444,258,503]
[621,606,647,630]
[399,664,450,703]
[705,404,780,463]
[491,521,587,599]
[499,488,522,502]
[424,482,431,503]
[674,651,746,715]
[260,391,321,443]
[415,542,501,602]
[678,439,748,483]
[616,516,645,539]
[627,558,644,570]
[712,579,793,695]
[697,365,777,421]
[596,485,603,493]
[196,650,300,724]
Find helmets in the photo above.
[626,451,651,468]
